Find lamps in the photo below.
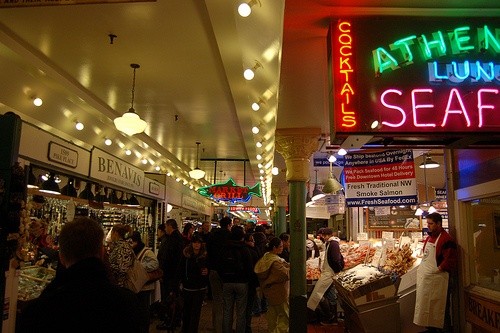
[418,153,445,169]
[54,175,61,183]
[189,142,205,180]
[322,162,343,195]
[114,64,147,136]
[414,200,437,216]
[41,172,50,181]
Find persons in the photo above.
[106,217,291,333]
[412,212,457,333]
[15,217,150,333]
[308,229,345,325]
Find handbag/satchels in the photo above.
[121,244,149,293]
[138,250,164,285]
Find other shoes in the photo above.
[321,312,334,322]
[261,306,268,314]
[321,320,338,326]
[156,322,171,330]
[253,310,262,316]
[202,300,207,307]
